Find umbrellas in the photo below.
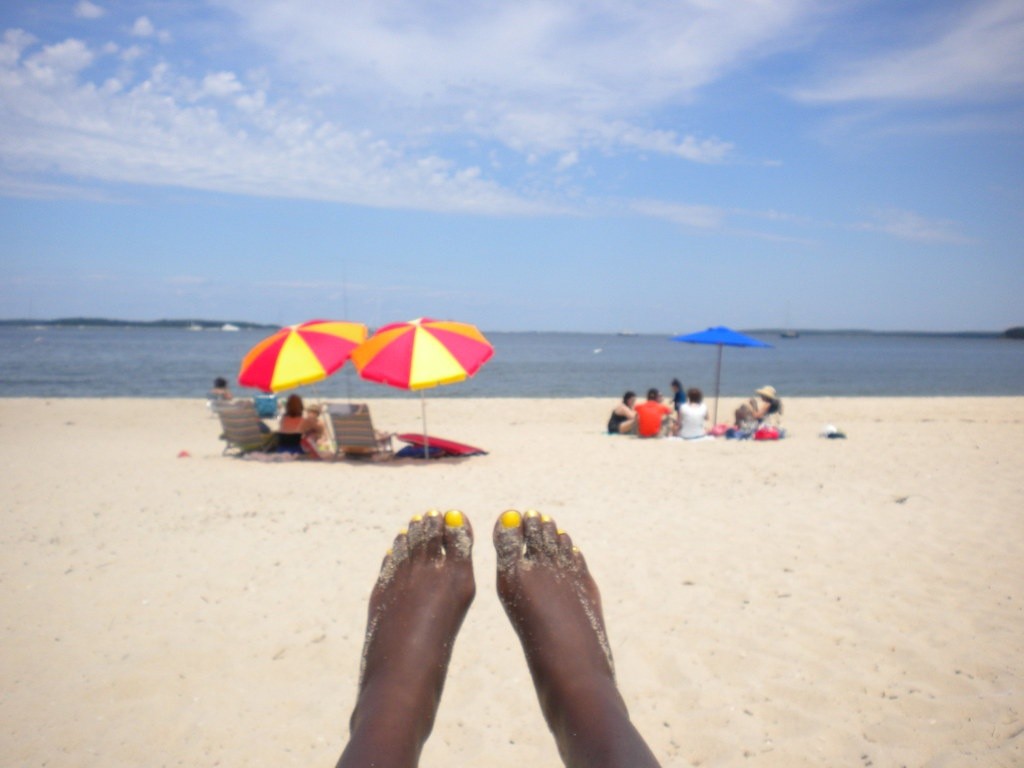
[237,320,369,448]
[670,326,776,428]
[349,317,494,459]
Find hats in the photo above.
[756,384,776,401]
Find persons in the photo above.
[606,376,784,441]
[208,376,329,446]
[328,510,663,768]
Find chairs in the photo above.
[212,393,395,461]
